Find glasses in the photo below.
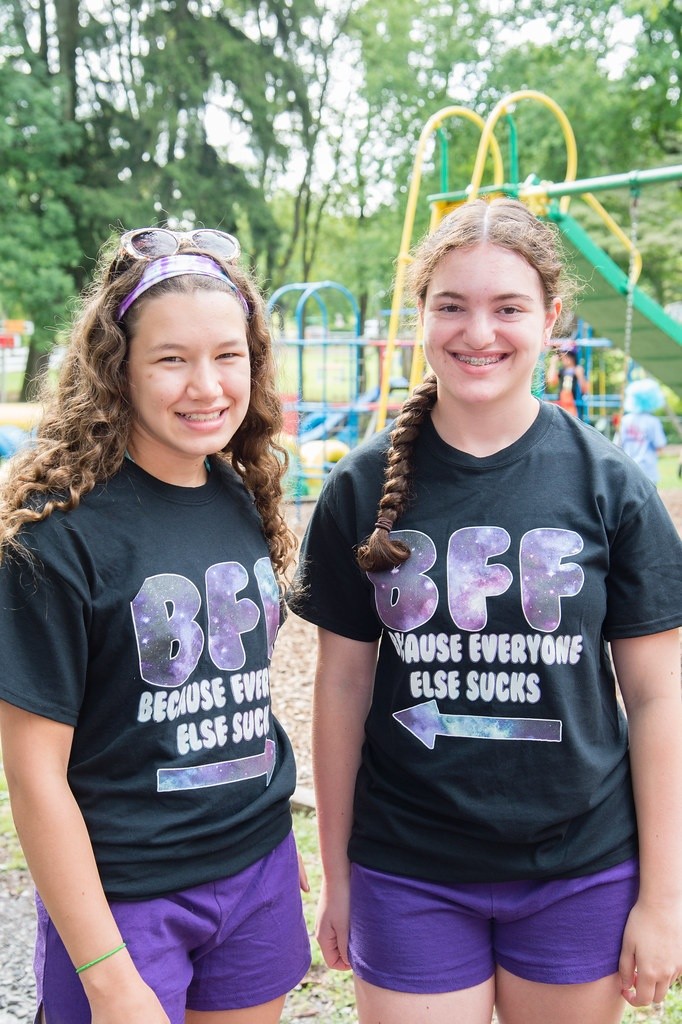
[108,228,241,284]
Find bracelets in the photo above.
[76,943,126,972]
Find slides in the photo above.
[538,199,681,398]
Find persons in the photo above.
[547,345,591,421]
[611,380,667,489]
[284,198,680,1024]
[0,223,312,1024]
